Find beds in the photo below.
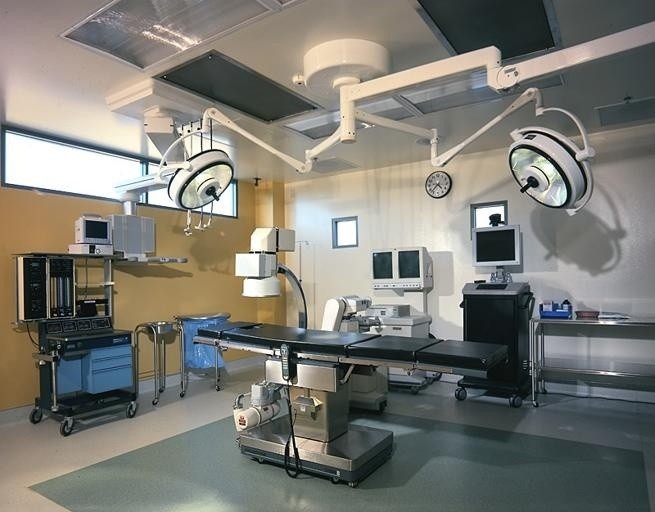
[193,323,508,378]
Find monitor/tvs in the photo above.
[395,246,432,283]
[74,219,112,245]
[472,225,520,266]
[369,248,395,283]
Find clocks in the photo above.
[425,171,452,199]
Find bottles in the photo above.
[561,298,572,310]
